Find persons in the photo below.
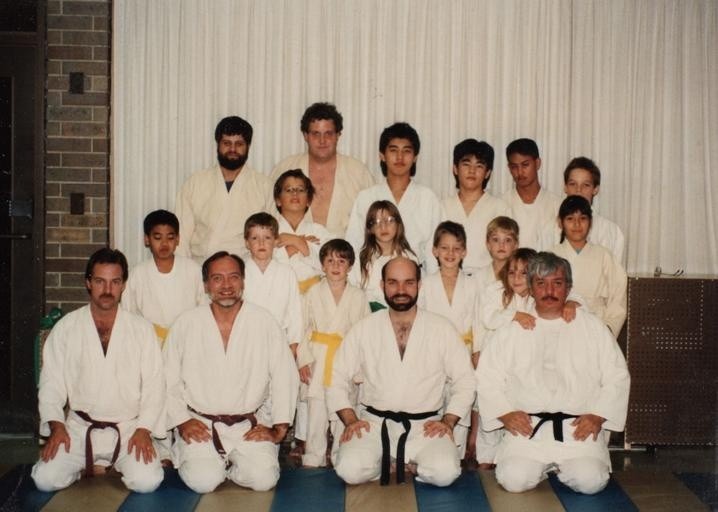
[121,208,204,353]
[163,252,300,494]
[31,248,168,493]
[269,101,375,241]
[241,123,632,494]
[173,116,275,267]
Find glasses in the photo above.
[373,216,395,224]
